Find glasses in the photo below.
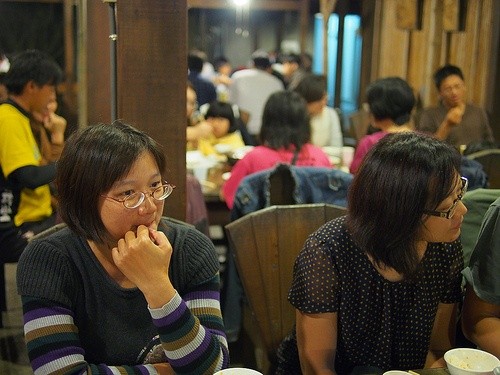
[98,178,177,209]
[419,176,469,219]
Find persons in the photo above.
[461,197,500,358]
[219,63,497,212]
[274,130,467,375]
[0,48,78,262]
[15,119,229,375]
[186,48,312,150]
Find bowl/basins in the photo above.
[212,367,263,375]
[444,348,500,375]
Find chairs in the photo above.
[351,110,372,141]
[268,162,296,206]
[224,203,349,361]
[467,150,500,190]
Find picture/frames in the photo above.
[442,0,461,33]
[394,0,419,32]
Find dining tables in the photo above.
[203,157,233,203]
